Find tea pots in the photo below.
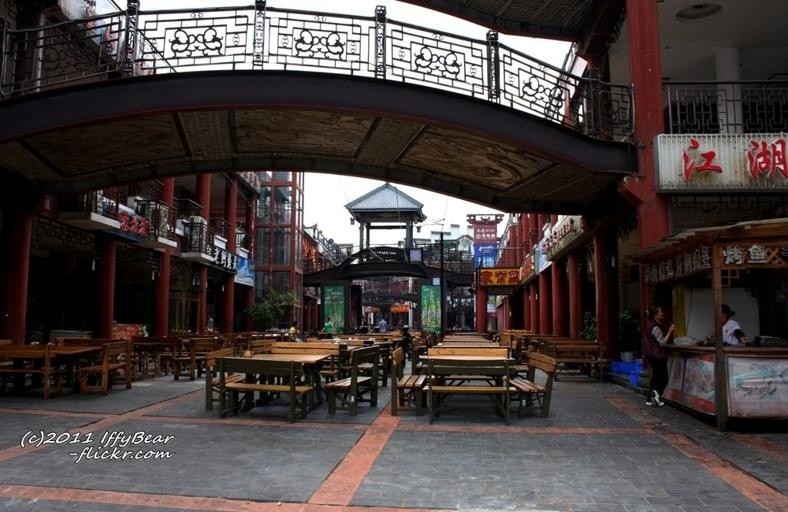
[241,349,255,358]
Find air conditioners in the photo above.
[188,214,207,253]
[145,200,169,239]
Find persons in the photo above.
[687,302,750,350]
[287,319,304,344]
[377,314,388,333]
[359,315,369,333]
[642,303,677,408]
[320,315,336,340]
[452,321,462,333]
[402,324,415,361]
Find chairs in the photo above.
[204,321,603,426]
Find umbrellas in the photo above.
[364,306,381,318]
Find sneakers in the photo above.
[645,389,664,407]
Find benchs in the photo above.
[0,325,205,400]
